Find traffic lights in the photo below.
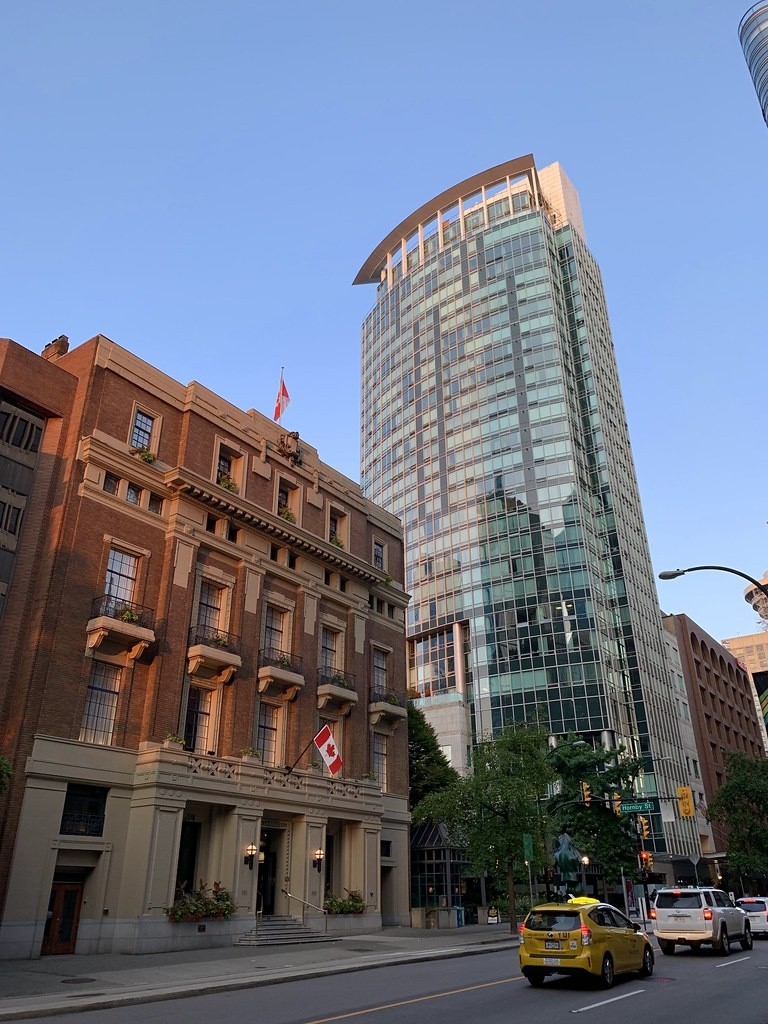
[646,852,654,869]
[613,792,622,815]
[583,781,591,807]
[639,817,650,839]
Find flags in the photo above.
[274,377,290,422]
[314,725,342,775]
[696,801,707,820]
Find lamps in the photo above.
[243,841,258,870]
[312,847,324,873]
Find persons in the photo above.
[650,888,657,903]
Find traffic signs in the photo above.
[620,802,655,813]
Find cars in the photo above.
[735,897,768,936]
[517,898,657,989]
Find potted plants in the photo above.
[307,761,324,778]
[361,770,380,786]
[162,733,187,751]
[323,890,365,915]
[241,746,262,766]
[165,880,239,924]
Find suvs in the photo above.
[651,885,754,957]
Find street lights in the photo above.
[632,756,672,914]
[536,741,586,898]
